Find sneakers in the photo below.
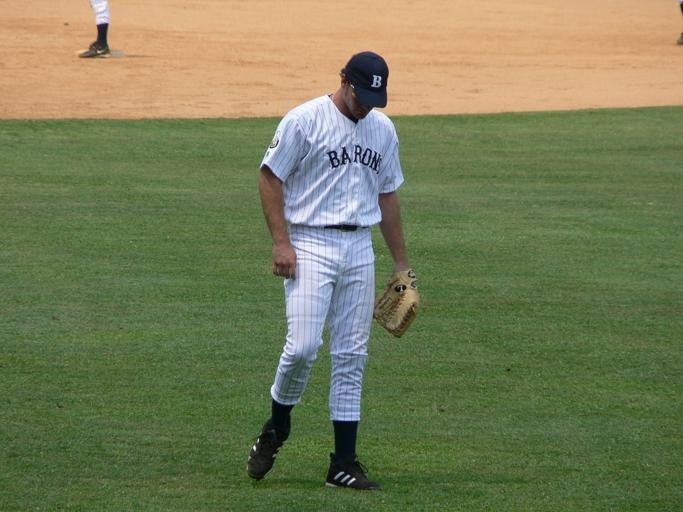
[323,451,381,490]
[77,40,111,58]
[246,414,292,482]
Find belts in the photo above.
[324,224,358,231]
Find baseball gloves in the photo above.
[374,269,420,338]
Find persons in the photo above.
[74,0,111,59]
[242,51,420,491]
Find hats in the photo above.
[341,50,389,109]
